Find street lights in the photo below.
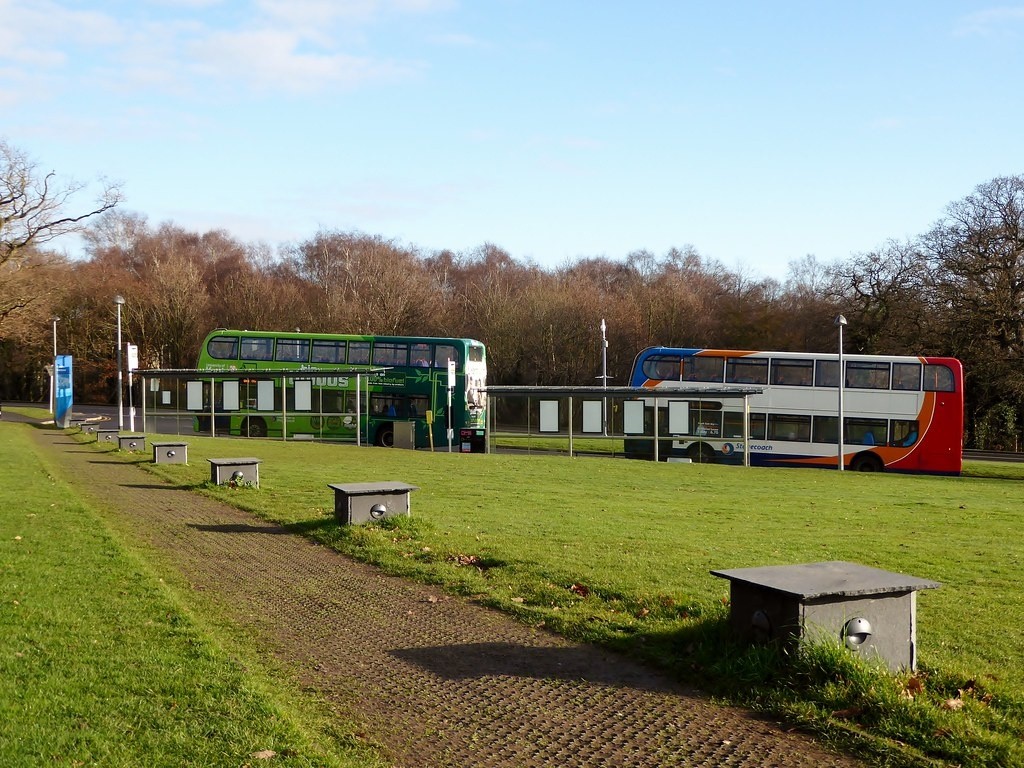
[112,294,126,431]
[836,317,848,470]
[48,315,61,414]
[600,319,609,435]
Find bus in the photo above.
[193,328,489,449]
[622,346,965,471]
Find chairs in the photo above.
[672,370,902,390]
[387,404,396,416]
[212,350,440,367]
[409,404,417,418]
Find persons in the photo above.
[687,372,698,380]
[411,399,418,416]
[755,376,760,383]
[915,378,918,388]
[899,424,918,446]
[732,375,737,381]
[710,373,718,381]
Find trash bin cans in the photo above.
[459,428,486,453]
